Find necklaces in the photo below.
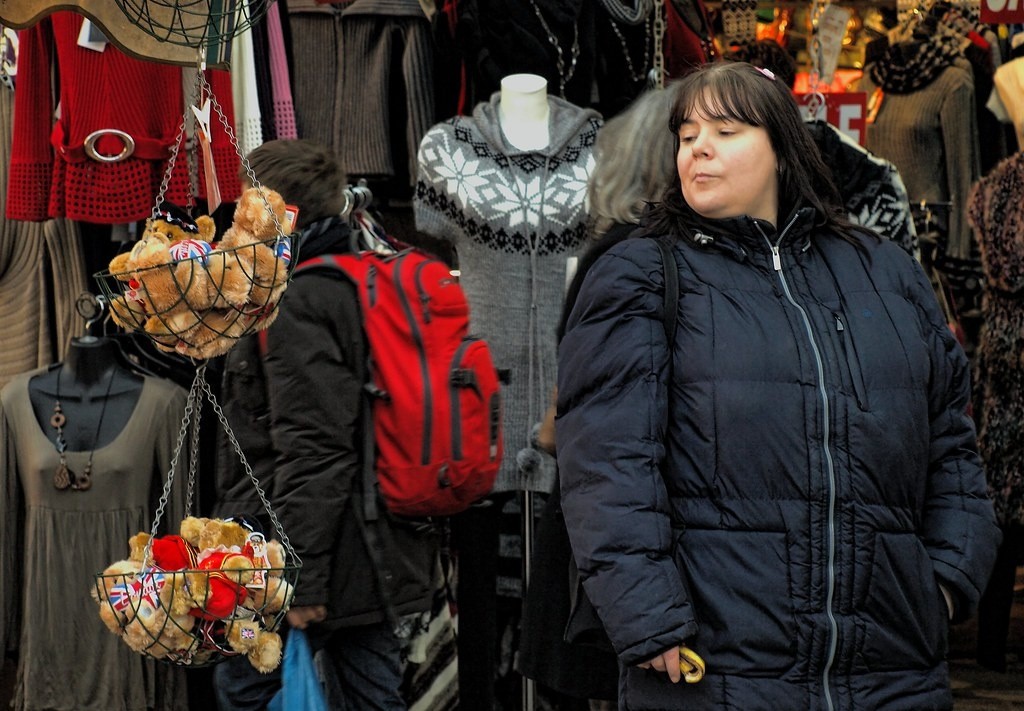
[48,358,120,491]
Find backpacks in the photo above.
[557,188,679,647]
[255,234,506,518]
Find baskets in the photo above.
[92,366,303,668]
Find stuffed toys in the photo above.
[86,516,300,675]
[108,187,292,361]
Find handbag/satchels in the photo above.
[265,626,329,711]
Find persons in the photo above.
[555,66,997,708]
[513,81,704,708]
[0,325,198,711]
[412,70,609,494]
[860,35,969,268]
[960,55,1024,580]
[221,132,453,708]
